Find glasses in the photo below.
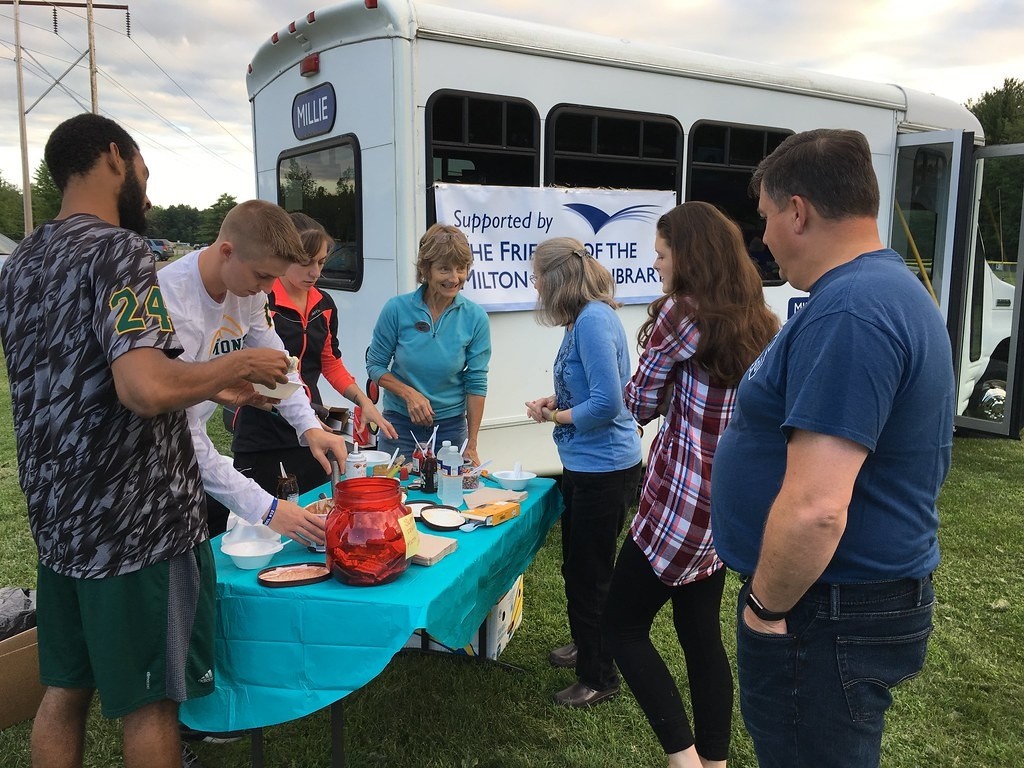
[530,273,545,284]
[425,232,470,244]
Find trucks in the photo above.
[246,0,1024,476]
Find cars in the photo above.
[146,240,175,261]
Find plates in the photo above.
[463,482,484,491]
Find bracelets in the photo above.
[263,497,278,527]
[552,409,564,426]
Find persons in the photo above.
[711,128,954,768]
[367,225,492,476]
[0,112,290,768]
[156,201,348,768]
[524,238,644,710]
[232,212,399,501]
[600,201,783,768]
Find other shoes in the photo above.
[179,724,242,743]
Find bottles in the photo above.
[345,452,366,479]
[277,475,299,505]
[324,476,419,586]
[412,440,463,508]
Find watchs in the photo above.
[744,585,787,621]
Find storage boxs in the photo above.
[0,627,46,731]
[401,573,524,661]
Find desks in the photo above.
[178,465,562,768]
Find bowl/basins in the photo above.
[304,498,334,552]
[462,465,480,489]
[360,450,391,466]
[493,470,537,490]
[252,381,308,399]
[220,520,283,570]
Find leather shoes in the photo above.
[550,642,579,664]
[554,681,619,709]
